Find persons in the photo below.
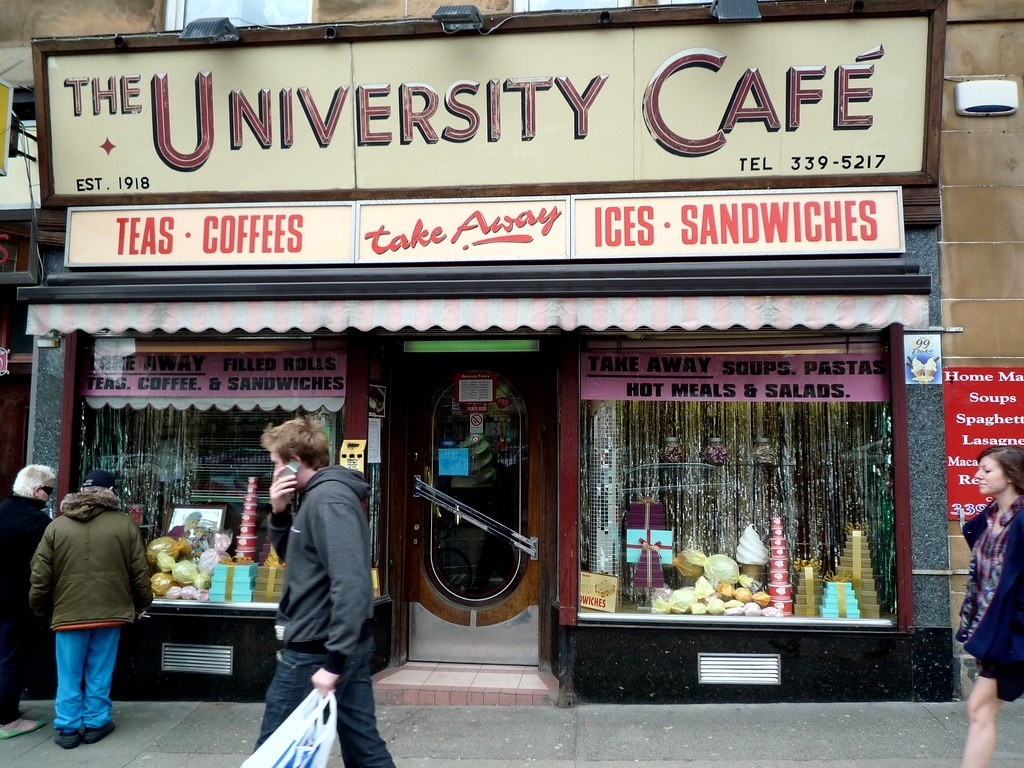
[29,469,152,749]
[253,417,397,768]
[0,464,58,739]
[954,445,1024,768]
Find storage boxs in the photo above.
[208,476,284,603]
[626,502,674,587]
[768,518,881,619]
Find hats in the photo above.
[81,469,120,496]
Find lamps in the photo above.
[709,0,762,23]
[177,16,242,43]
[432,5,484,34]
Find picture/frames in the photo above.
[162,503,227,569]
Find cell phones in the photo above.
[278,459,301,481]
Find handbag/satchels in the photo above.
[239,688,338,768]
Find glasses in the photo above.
[40,486,54,496]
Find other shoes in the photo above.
[0,720,46,739]
[54,731,80,749]
[83,720,116,744]
